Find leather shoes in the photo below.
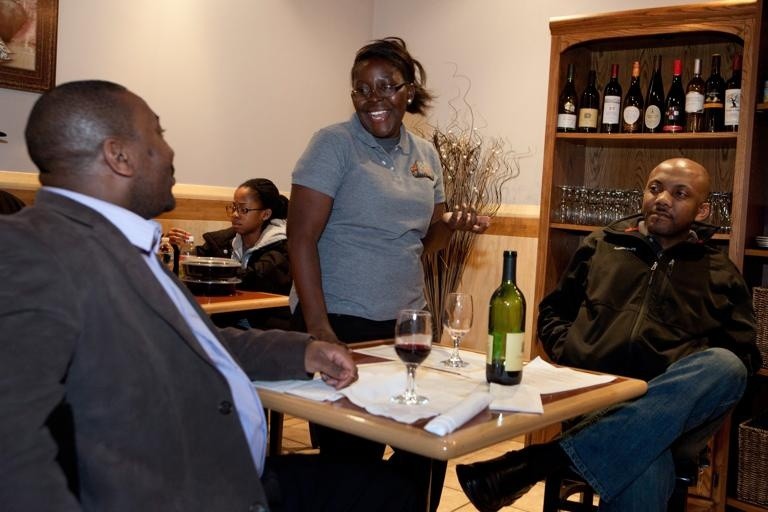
[456,450,550,512]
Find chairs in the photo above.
[538,467,692,509]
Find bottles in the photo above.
[178,236,196,280]
[661,59,685,133]
[643,54,665,133]
[724,55,742,132]
[577,70,600,133]
[622,61,644,133]
[486,251,526,385]
[685,58,706,133]
[557,63,578,132]
[158,237,174,272]
[600,64,622,133]
[704,53,725,132]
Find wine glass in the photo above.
[390,309,431,407]
[707,191,732,232]
[551,183,643,227]
[438,292,473,370]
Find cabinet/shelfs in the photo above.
[524,0,766,510]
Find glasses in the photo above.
[353,82,407,99]
[233,205,264,214]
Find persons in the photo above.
[454,156,762,512]
[0,78,359,512]
[285,35,494,511]
[162,174,291,332]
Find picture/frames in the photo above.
[0,0,62,96]
